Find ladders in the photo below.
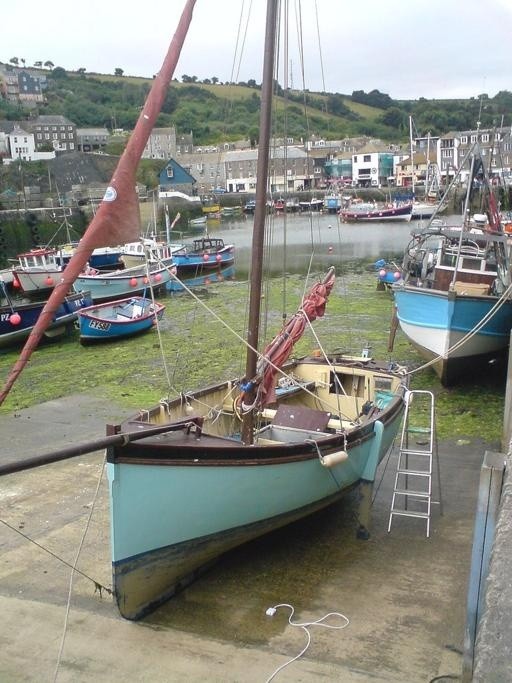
[387,390,444,538]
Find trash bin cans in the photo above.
[378,184,382,189]
